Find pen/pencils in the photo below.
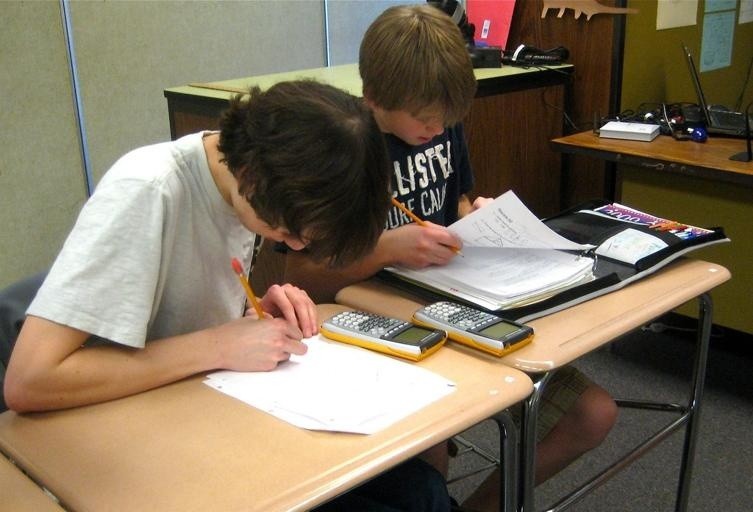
[391,197,465,257]
[231,256,266,320]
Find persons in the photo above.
[3,80,459,512]
[255,2,620,512]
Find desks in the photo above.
[326,247,731,510]
[0,299,539,511]
[158,50,576,313]
[544,111,751,360]
[0,448,70,512]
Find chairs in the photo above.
[0,263,111,418]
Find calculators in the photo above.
[320,310,448,362]
[411,300,535,358]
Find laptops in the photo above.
[682,41,753,138]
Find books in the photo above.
[379,190,600,319]
[599,120,662,142]
